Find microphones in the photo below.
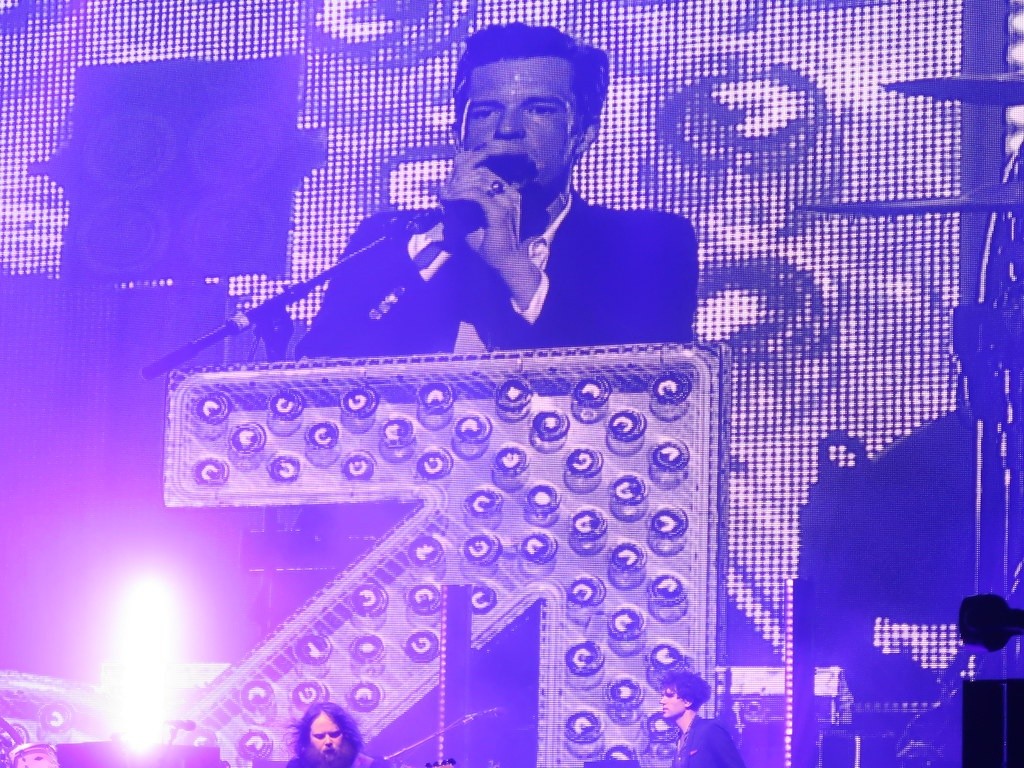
[169,719,195,732]
[449,155,530,222]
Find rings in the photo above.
[486,180,504,198]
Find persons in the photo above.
[283,699,380,768]
[656,671,746,768]
[296,20,700,347]
